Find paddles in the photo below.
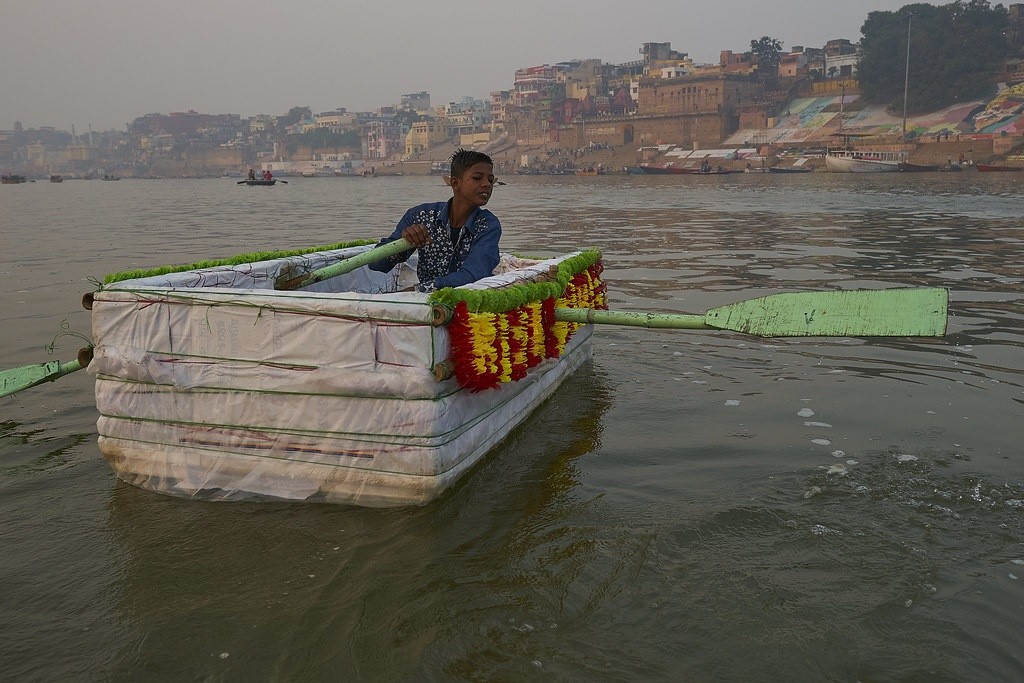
[1,237,409,397]
[497,181,507,185]
[237,181,246,184]
[272,178,288,184]
[554,285,950,340]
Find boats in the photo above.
[0,178,36,184]
[824,153,900,173]
[744,167,769,173]
[83,232,611,512]
[770,166,812,174]
[639,165,693,175]
[50,178,64,183]
[101,178,120,182]
[975,162,1024,171]
[898,162,939,172]
[245,178,276,185]
[940,164,962,172]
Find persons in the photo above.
[700,159,709,169]
[575,167,604,173]
[955,131,960,142]
[368,148,502,294]
[264,171,273,181]
[936,131,941,142]
[260,170,266,180]
[248,169,256,180]
[945,130,948,142]
[959,152,963,164]
[943,153,951,168]
